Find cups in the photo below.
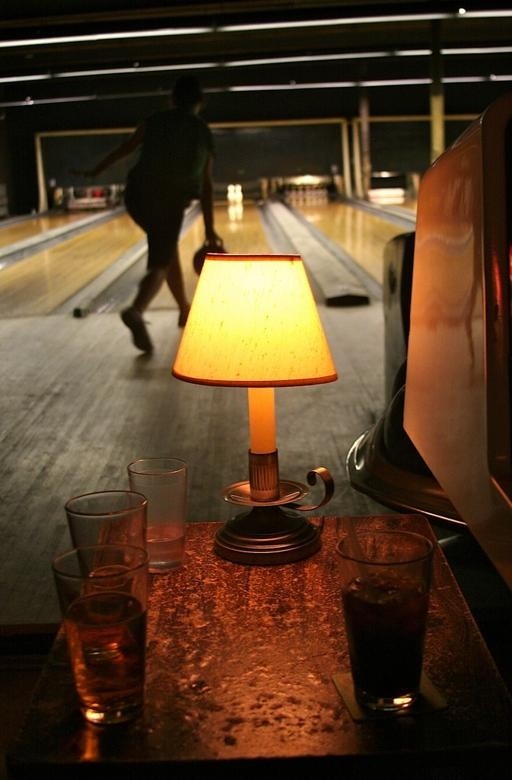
[337,530,435,717]
[51,458,187,728]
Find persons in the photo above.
[77,78,223,352]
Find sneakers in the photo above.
[119,305,154,353]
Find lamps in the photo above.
[171,252,339,567]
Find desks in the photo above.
[8,514,512,765]
